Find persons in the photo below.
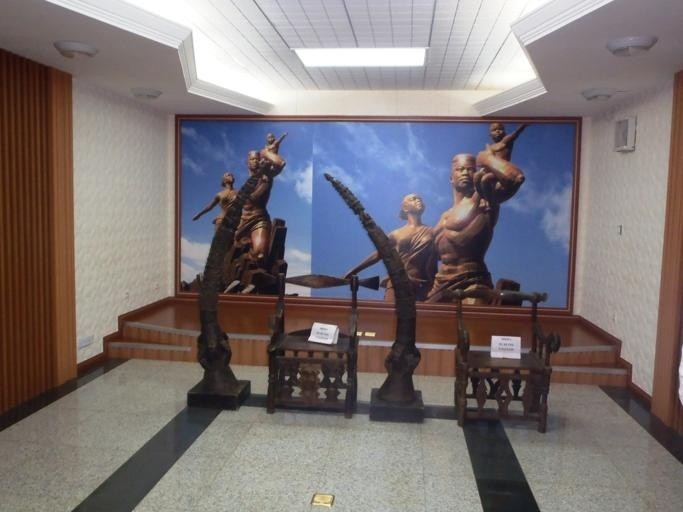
[424,143,525,306]
[473,121,530,211]
[192,131,287,294]
[343,193,441,304]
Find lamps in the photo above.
[130,87,162,102]
[53,41,98,60]
[607,34,659,58]
[582,88,614,103]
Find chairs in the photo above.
[267,272,358,418]
[447,287,561,433]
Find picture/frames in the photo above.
[174,113,582,316]
